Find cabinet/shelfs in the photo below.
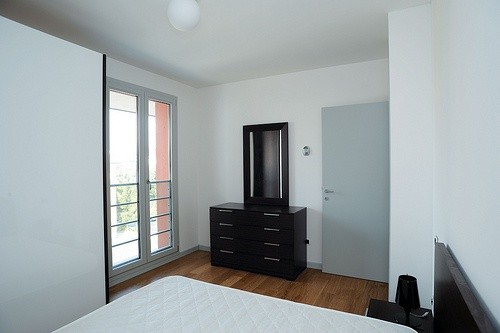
[209,202,307,282]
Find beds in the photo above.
[50,238,495,333]
